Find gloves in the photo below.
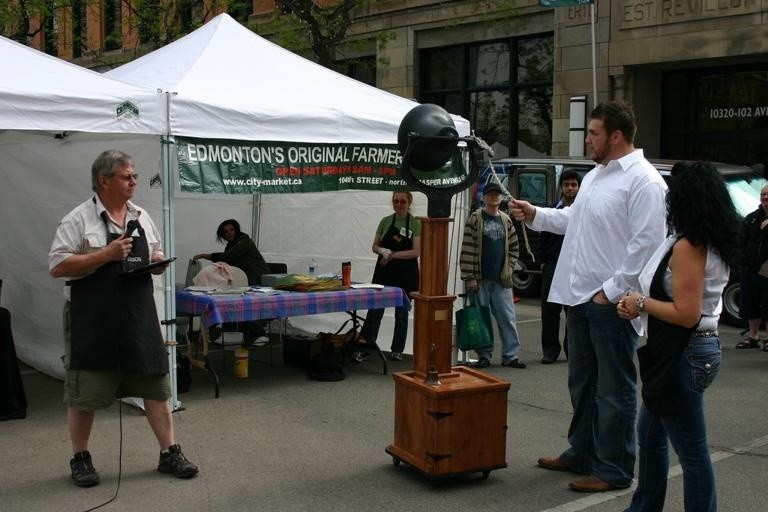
[378,248,392,260]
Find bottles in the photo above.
[309,259,318,276]
[342,260,351,288]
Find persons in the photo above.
[533,168,582,364]
[351,191,422,362]
[736,184,768,352]
[506,98,672,494]
[46,147,199,487]
[459,183,527,368]
[191,219,272,347]
[614,159,755,512]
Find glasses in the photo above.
[392,199,408,205]
[114,173,138,180]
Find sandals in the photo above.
[736,336,760,349]
[763,338,768,352]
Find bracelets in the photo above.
[636,293,647,312]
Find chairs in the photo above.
[242,263,287,344]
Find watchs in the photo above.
[601,288,610,302]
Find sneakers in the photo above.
[70,451,99,487]
[356,352,368,362]
[392,352,402,361]
[157,444,198,478]
[541,355,557,364]
[475,357,490,368]
[501,358,527,369]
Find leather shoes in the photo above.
[537,456,567,471]
[568,475,630,492]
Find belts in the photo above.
[693,330,719,339]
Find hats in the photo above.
[483,183,502,196]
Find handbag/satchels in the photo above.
[336,319,364,362]
[456,290,494,352]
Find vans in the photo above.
[474,157,767,330]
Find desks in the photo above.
[176,278,413,399]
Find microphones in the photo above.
[122,220,137,240]
